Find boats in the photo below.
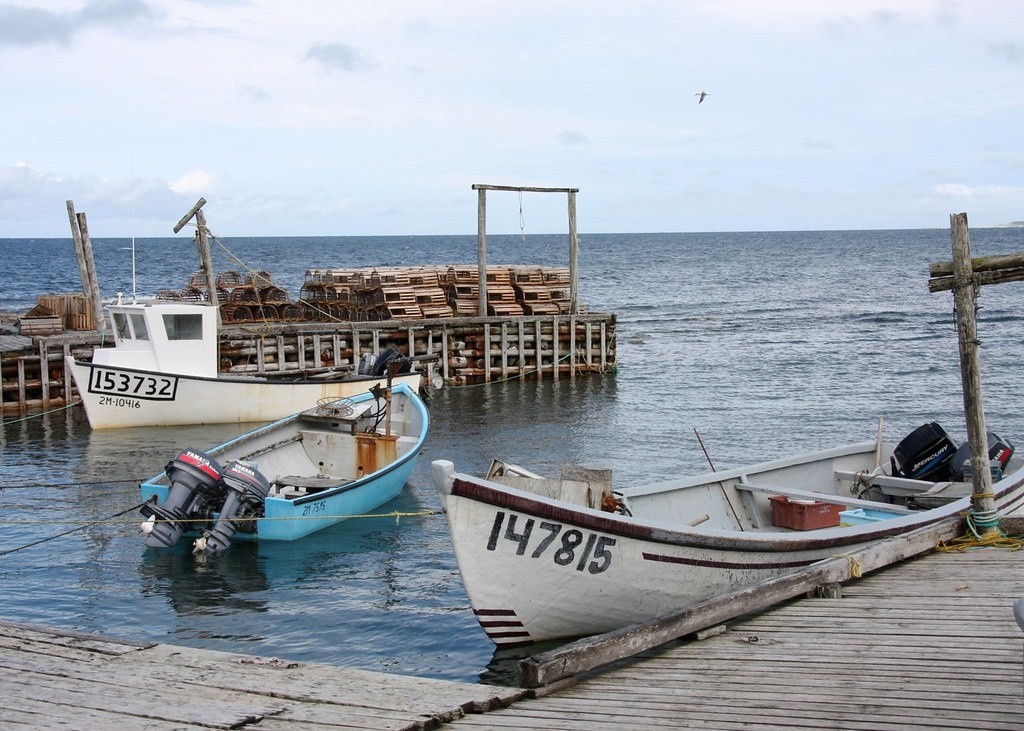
[138,380,432,568]
[63,226,440,429]
[429,418,1024,647]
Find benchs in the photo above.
[735,482,929,514]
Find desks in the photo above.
[301,405,372,435]
[275,475,354,494]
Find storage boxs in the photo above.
[889,422,1015,481]
[839,508,906,528]
[768,491,846,530]
